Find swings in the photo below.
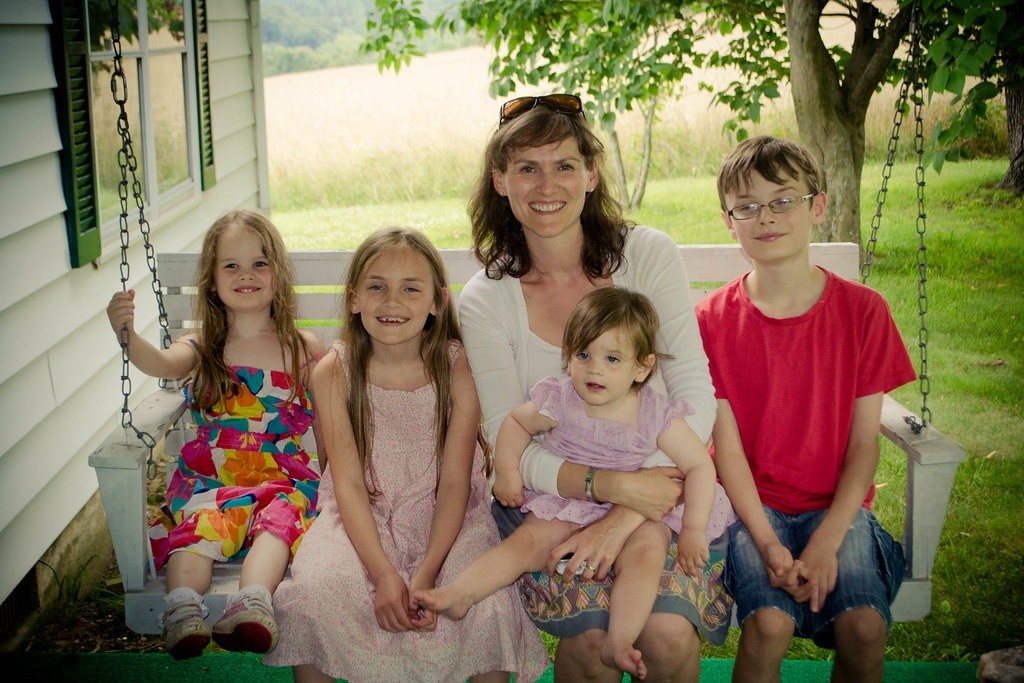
[93,0,958,639]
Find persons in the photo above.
[459,96,720,683]
[412,287,737,680]
[694,137,917,683]
[261,231,550,683]
[107,211,329,661]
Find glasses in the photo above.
[499,94,587,129]
[727,192,814,220]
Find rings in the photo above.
[586,565,596,572]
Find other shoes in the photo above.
[163,599,211,661]
[211,595,280,654]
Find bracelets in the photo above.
[584,467,607,505]
